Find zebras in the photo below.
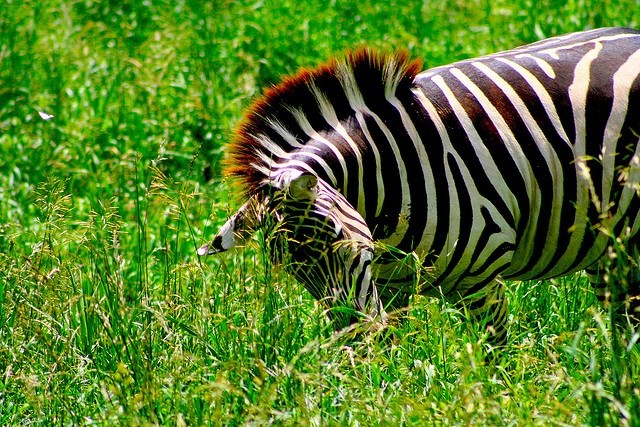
[198,25,640,398]
